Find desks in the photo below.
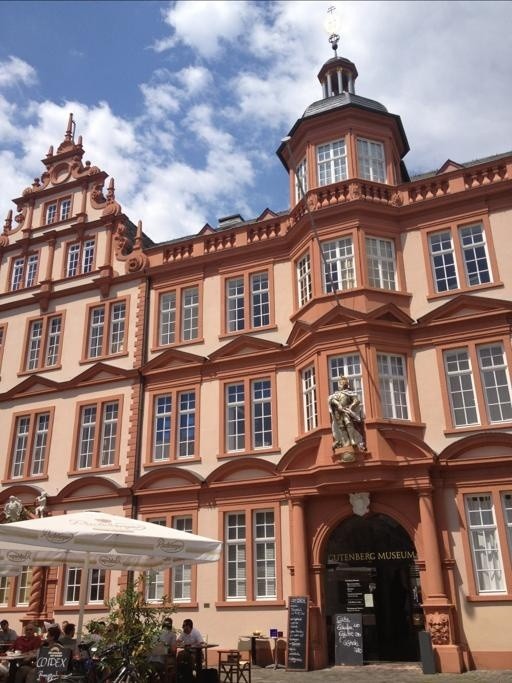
[241,636,268,668]
[265,636,286,670]
[0,639,219,683]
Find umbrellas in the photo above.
[1,508,224,651]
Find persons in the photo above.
[1,616,208,683]
[35,490,48,518]
[5,494,22,517]
[326,375,365,447]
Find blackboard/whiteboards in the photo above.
[286,596,309,671]
[335,614,363,666]
[36,647,71,673]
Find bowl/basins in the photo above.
[252,633,260,636]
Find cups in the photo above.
[7,652,14,656]
[15,650,22,655]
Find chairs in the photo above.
[216,650,251,683]
